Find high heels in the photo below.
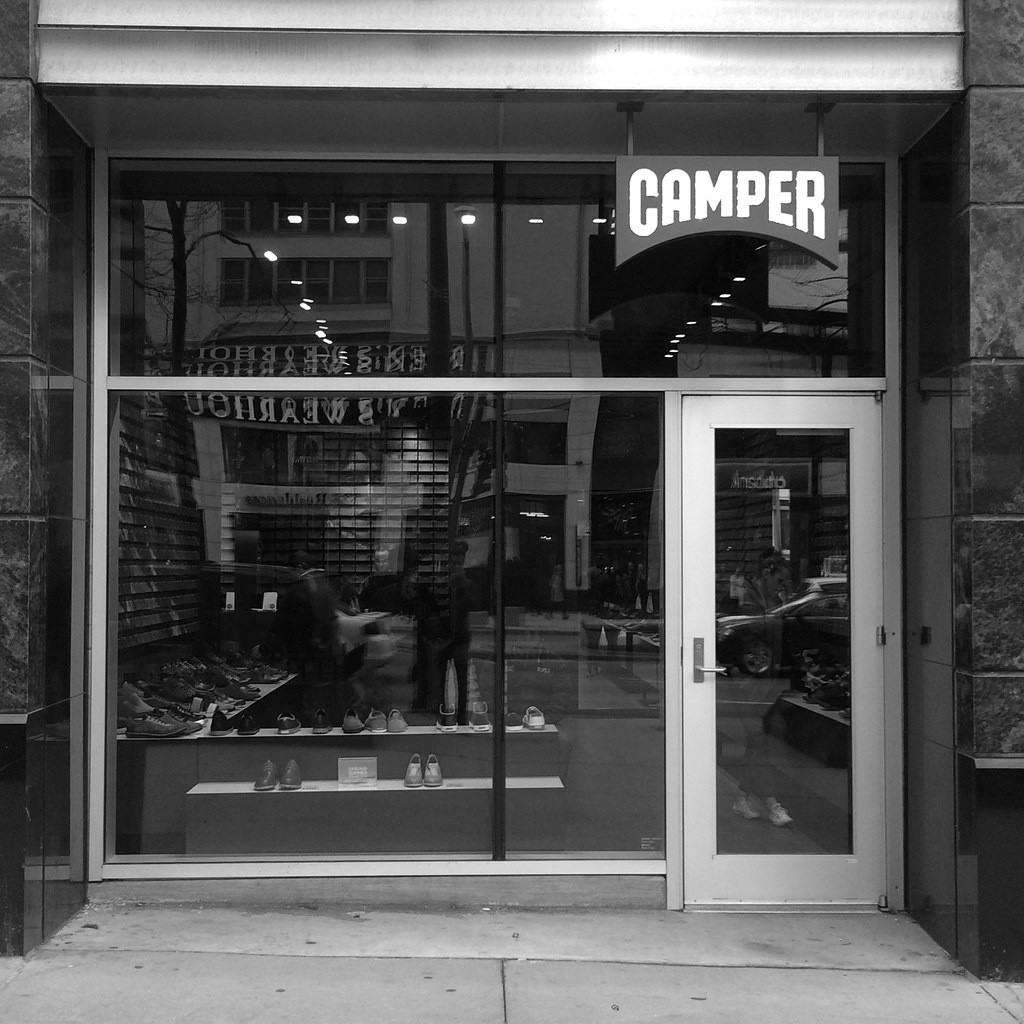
[404,752,422,787]
[423,753,442,786]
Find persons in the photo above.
[274,549,337,722]
[357,537,471,724]
[580,554,661,676]
[726,549,794,827]
[546,565,569,620]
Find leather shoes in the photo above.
[253,759,279,791]
[279,759,302,789]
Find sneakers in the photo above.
[469,701,492,731]
[436,703,459,732]
[312,708,333,734]
[733,799,761,820]
[767,802,795,827]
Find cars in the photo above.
[335,607,395,670]
[714,590,850,675]
[717,592,851,676]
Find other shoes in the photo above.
[117,653,289,738]
[801,669,852,719]
[505,712,523,731]
[584,666,601,677]
[365,706,387,733]
[237,712,259,735]
[387,708,406,732]
[211,711,234,736]
[276,712,301,734]
[522,706,546,730]
[342,707,365,733]
